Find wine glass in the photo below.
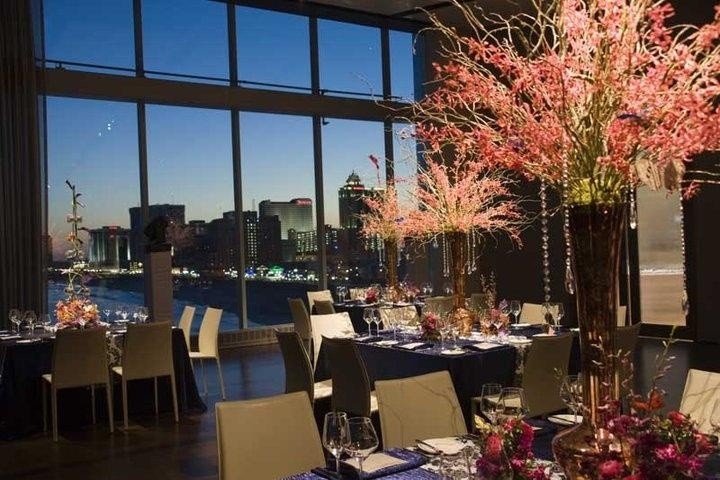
[322,410,352,479]
[347,414,380,479]
[0,284,588,450]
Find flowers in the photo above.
[52,179,102,329]
[350,1,719,246]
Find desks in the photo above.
[290,411,720,480]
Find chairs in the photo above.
[41,328,115,443]
[678,369,719,438]
[178,304,227,403]
[215,391,327,479]
[110,320,183,434]
[274,283,642,420]
[374,370,469,450]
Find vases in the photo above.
[549,199,655,480]
[382,238,406,304]
[445,230,474,337]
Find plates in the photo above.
[417,437,475,457]
